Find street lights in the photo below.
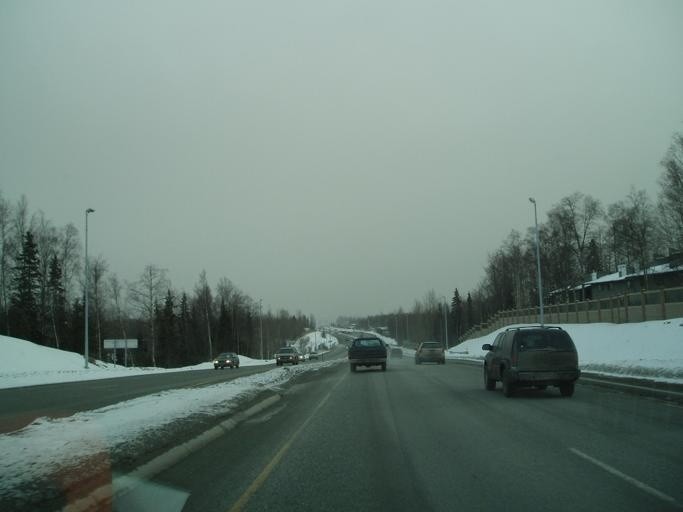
[83,207,96,369]
[527,197,546,328]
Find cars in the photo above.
[309,352,318,360]
[346,336,389,371]
[390,348,404,360]
[213,352,239,370]
[416,341,445,366]
[276,346,299,366]
[482,324,581,398]
[298,352,306,362]
